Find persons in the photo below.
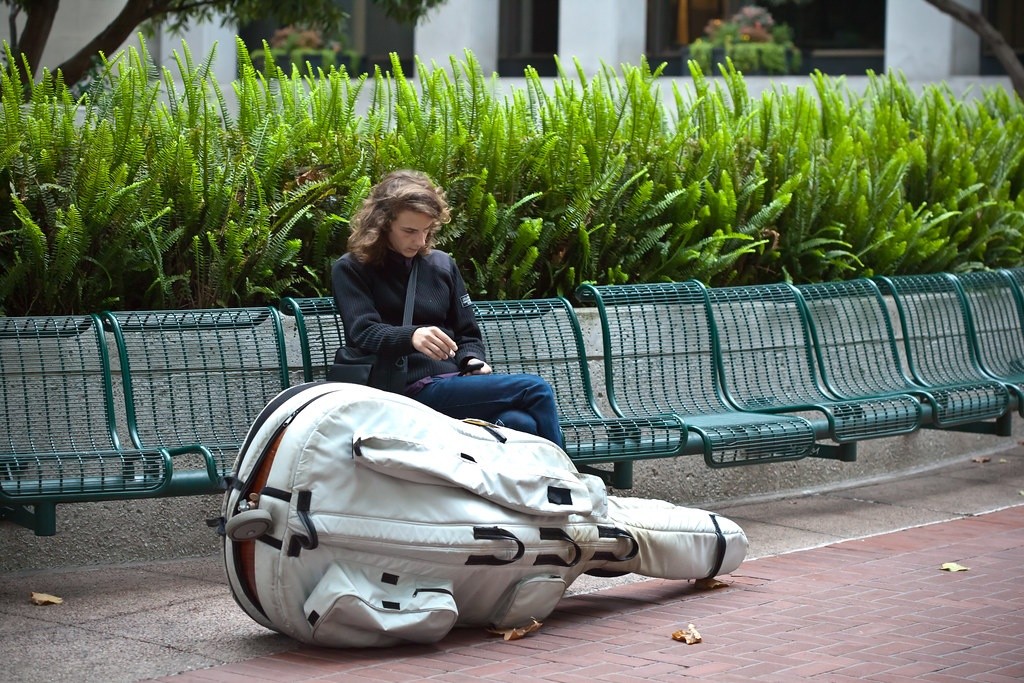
[332,170,563,449]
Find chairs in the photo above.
[0,265,1024,537]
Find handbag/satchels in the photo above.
[326,346,407,396]
